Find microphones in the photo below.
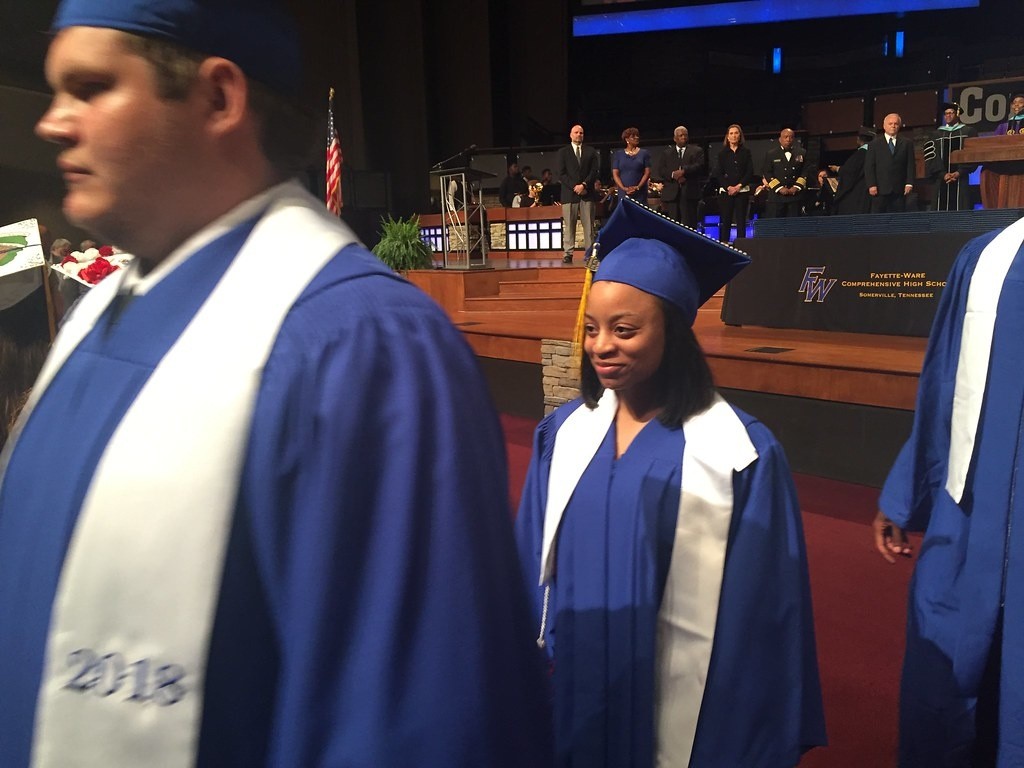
[1011,107,1024,135]
[460,144,476,155]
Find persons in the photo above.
[713,124,752,242]
[515,197,829,768]
[866,114,916,213]
[871,212,1024,768]
[448,174,476,212]
[0,0,557,768]
[0,217,60,458]
[613,128,650,208]
[994,94,1024,136]
[923,103,978,211]
[501,162,563,206]
[762,129,807,218]
[39,224,96,265]
[816,127,876,216]
[658,125,704,228]
[556,124,602,261]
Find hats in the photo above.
[570,194,752,384]
[858,125,877,142]
[36,2,310,104]
[1008,90,1024,103]
[943,102,965,116]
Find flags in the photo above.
[326,100,344,220]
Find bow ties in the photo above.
[782,147,792,153]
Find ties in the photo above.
[888,138,896,155]
[576,146,582,166]
[679,148,683,158]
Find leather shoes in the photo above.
[563,254,572,263]
